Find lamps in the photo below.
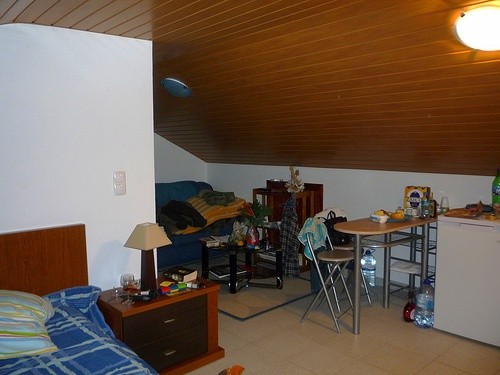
[123,222,172,292]
[454,1,500,51]
[160,78,192,97]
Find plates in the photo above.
[443,208,481,217]
[388,214,409,222]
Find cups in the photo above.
[441,196,449,213]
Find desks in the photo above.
[199,232,282,293]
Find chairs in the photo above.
[299,210,377,334]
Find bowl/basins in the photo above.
[370,214,380,222]
[378,216,388,223]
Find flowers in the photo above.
[240,202,271,226]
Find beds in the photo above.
[0,225,161,375]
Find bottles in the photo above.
[403,292,415,323]
[492,174,500,212]
[413,279,434,328]
[361,250,376,287]
[420,192,437,219]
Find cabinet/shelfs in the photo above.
[332,217,436,333]
[434,213,500,347]
[252,180,323,274]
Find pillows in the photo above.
[0,287,59,360]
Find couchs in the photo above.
[155,181,236,272]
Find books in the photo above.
[210,265,247,279]
[166,266,197,282]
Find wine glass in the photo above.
[120,274,136,305]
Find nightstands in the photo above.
[96,275,225,375]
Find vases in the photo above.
[246,225,259,245]
